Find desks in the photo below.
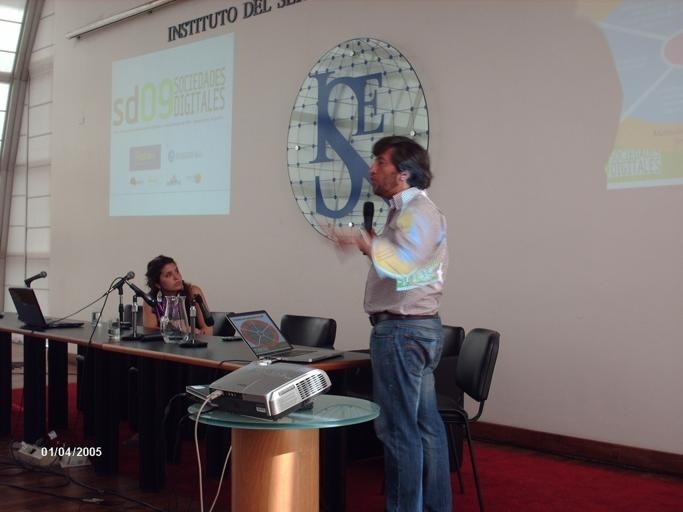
[184,395,382,511]
[1,309,370,494]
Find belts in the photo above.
[372,311,438,324]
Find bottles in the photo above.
[159,295,190,345]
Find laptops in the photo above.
[8,288,86,329]
[225,310,344,364]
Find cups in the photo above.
[107,318,120,342]
[89,310,101,327]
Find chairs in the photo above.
[126,316,143,327]
[346,323,499,511]
[207,311,238,338]
[280,315,338,351]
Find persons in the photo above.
[323,135,454,512]
[143,255,214,337]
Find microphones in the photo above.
[125,280,158,308]
[105,272,136,293]
[195,293,215,327]
[362,201,374,254]
[25,272,47,284]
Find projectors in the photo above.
[208,361,331,421]
